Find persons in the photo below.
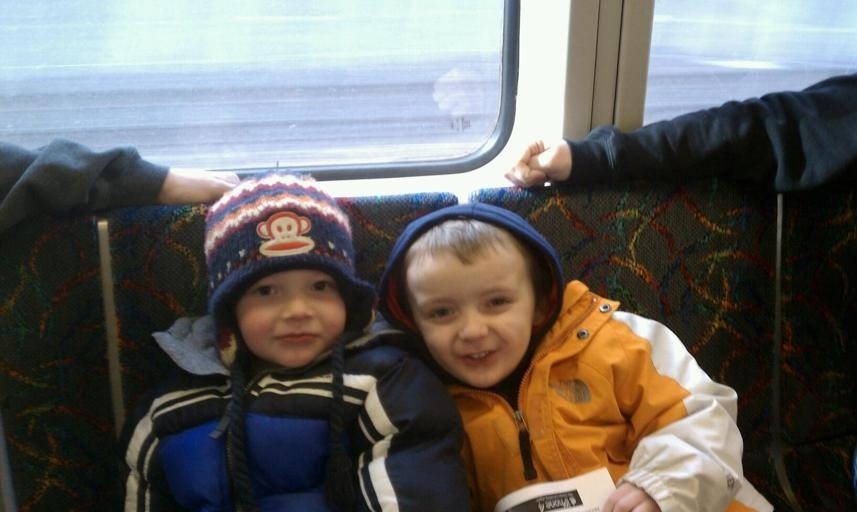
[0,136,240,234]
[506,74,851,194]
[117,171,471,512]
[376,203,777,512]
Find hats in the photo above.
[204,171,375,330]
[377,203,564,337]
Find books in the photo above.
[494,466,618,511]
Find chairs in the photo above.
[471,178,785,510]
[107,190,461,450]
[1,209,116,509]
[772,179,856,511]
